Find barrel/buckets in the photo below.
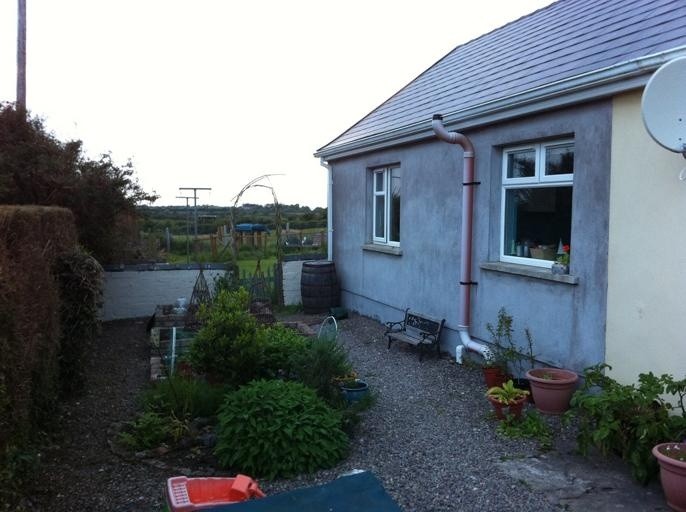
[301,261,341,315]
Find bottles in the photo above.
[556,239,568,261]
[510,239,530,257]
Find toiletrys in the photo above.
[511,239,530,257]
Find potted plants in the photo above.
[335,379,369,406]
[650,442,686,512]
[476,306,577,430]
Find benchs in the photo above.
[382,307,445,363]
